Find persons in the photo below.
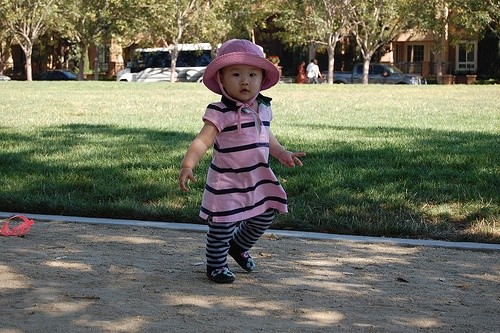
[299,59,323,84]
[179,40,306,283]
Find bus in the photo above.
[115,43,213,83]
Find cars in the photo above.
[22,68,88,81]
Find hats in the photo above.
[203,39,280,95]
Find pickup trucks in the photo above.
[321,62,428,85]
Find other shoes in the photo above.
[206,266,236,283]
[227,239,256,272]
